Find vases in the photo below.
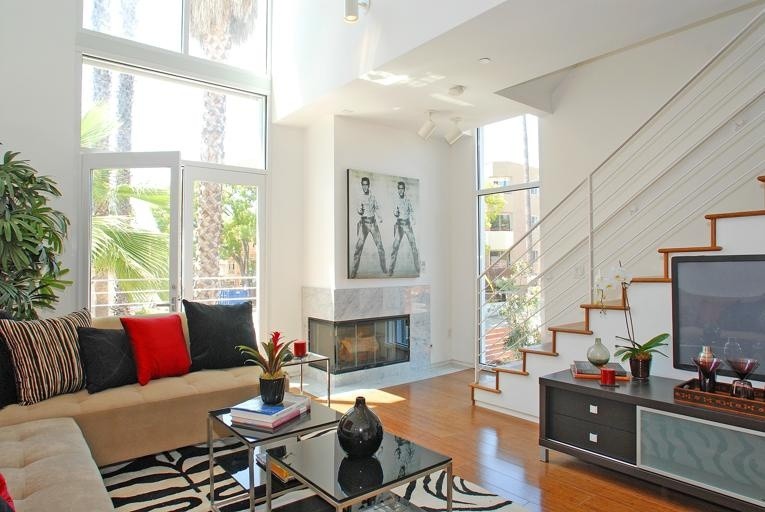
[259,379,289,403]
[588,338,612,369]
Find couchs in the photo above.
[2,418,116,512]
[0,300,285,468]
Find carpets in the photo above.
[95,400,520,509]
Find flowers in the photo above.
[238,333,306,379]
[586,264,670,363]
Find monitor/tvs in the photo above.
[670,254,765,383]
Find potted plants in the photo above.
[616,334,671,380]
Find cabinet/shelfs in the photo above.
[545,382,639,462]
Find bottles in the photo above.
[586,337,610,369]
[696,345,716,393]
[723,337,744,359]
[338,454,383,498]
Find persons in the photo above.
[383,182,419,275]
[350,176,388,277]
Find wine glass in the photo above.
[725,357,758,382]
[690,356,723,393]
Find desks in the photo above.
[270,346,331,409]
[208,404,453,511]
[539,363,765,509]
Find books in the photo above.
[231,395,310,429]
[571,359,631,381]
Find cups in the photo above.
[599,367,616,385]
[731,379,754,401]
[294,338,307,357]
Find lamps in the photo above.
[416,113,434,142]
[444,121,467,149]
[344,2,372,21]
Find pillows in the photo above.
[2,309,96,403]
[78,327,137,395]
[183,300,263,364]
[120,316,191,387]
